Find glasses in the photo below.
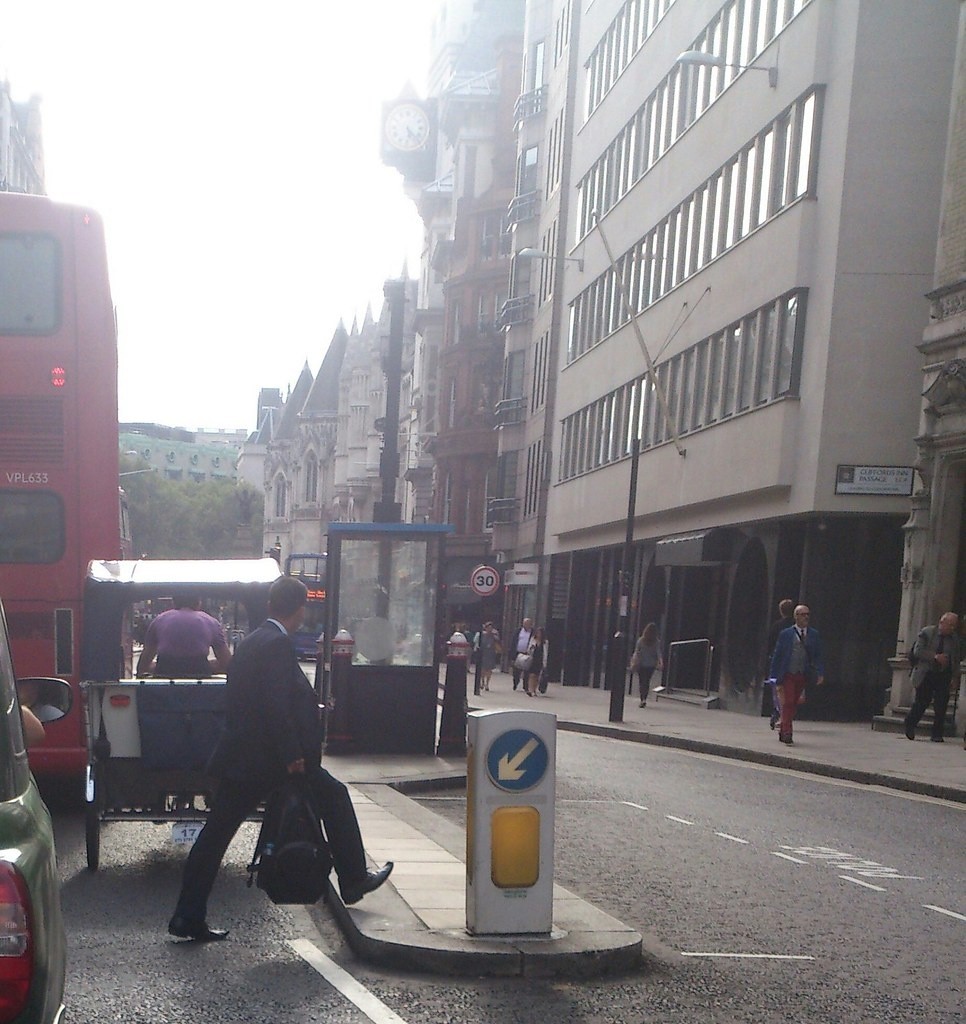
[795,612,810,617]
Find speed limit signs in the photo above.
[470,565,500,597]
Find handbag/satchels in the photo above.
[630,652,642,674]
[538,672,548,693]
[514,653,532,672]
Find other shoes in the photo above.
[639,700,645,709]
[341,860,394,906]
[779,732,793,746]
[485,685,489,692]
[513,683,517,690]
[527,691,533,697]
[931,736,943,743]
[168,917,230,941]
[770,720,781,731]
[904,719,915,741]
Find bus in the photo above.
[283,551,331,661]
[0,190,141,801]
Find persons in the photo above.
[134,614,152,646]
[510,618,548,697]
[448,621,500,691]
[904,612,961,742]
[170,577,394,942]
[635,623,661,708]
[22,705,45,745]
[19,681,64,721]
[137,595,232,680]
[768,600,823,744]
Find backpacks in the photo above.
[247,773,333,904]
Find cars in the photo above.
[0,598,76,1024]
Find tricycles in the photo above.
[75,554,285,874]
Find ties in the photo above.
[800,630,804,639]
[937,635,945,653]
